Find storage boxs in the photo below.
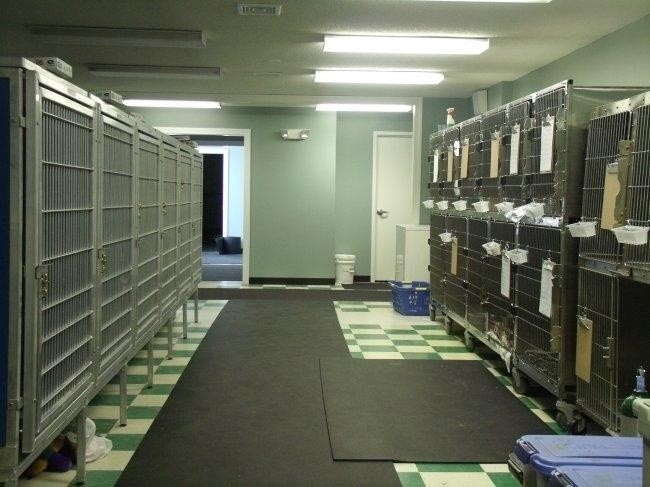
[507,434,643,487]
[393,288,430,316]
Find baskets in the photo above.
[391,281,430,316]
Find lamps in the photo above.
[314,103,413,113]
[314,68,444,86]
[322,35,489,55]
[122,99,221,110]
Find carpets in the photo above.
[319,357,559,463]
[115,301,403,486]
[188,288,393,302]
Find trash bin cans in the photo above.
[508,435,644,486]
